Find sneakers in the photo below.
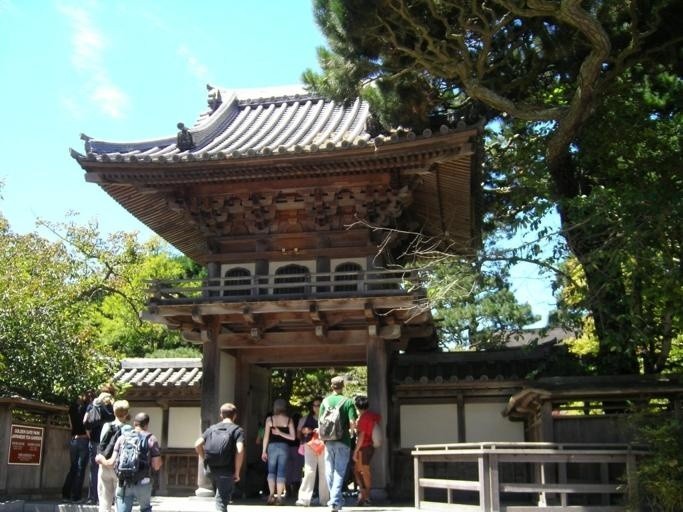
[61,497,97,506]
[327,502,339,512]
[354,494,372,507]
[295,500,310,507]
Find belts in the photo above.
[71,434,87,441]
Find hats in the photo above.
[330,376,344,389]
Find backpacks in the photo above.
[203,423,241,468]
[95,421,127,460]
[317,396,349,442]
[112,430,153,488]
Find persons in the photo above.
[96,399,133,512]
[62,391,91,501]
[82,384,117,504]
[94,411,164,512]
[255,374,382,512]
[194,402,246,512]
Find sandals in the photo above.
[267,495,289,507]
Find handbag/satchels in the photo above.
[83,404,102,430]
[370,421,384,450]
[297,432,306,456]
